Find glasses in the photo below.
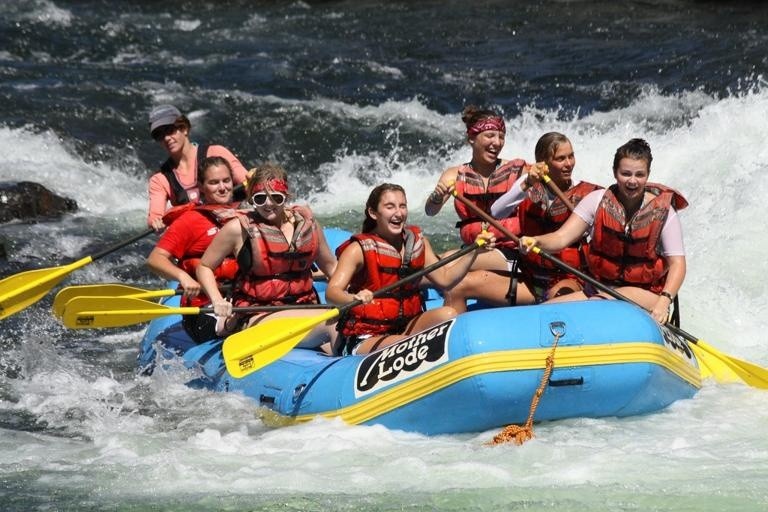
[253,192,286,208]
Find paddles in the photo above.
[0,180,247,319]
[541,173,743,383]
[446,181,713,380]
[524,244,768,393]
[63,295,337,329]
[222,236,485,378]
[53,284,233,319]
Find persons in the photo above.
[196,164,338,338]
[144,104,248,235]
[419,105,532,293]
[325,185,456,356]
[147,157,248,344]
[444,132,607,314]
[519,138,690,326]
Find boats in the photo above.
[135,225,701,438]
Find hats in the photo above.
[148,105,181,133]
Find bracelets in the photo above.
[430,191,443,204]
[660,290,673,304]
